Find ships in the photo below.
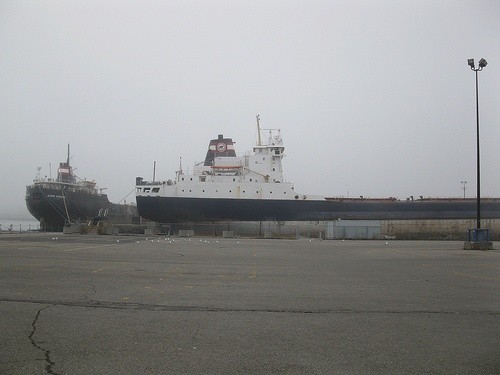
[133,106,328,200]
[25,140,140,233]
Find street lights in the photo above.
[467,56,488,228]
